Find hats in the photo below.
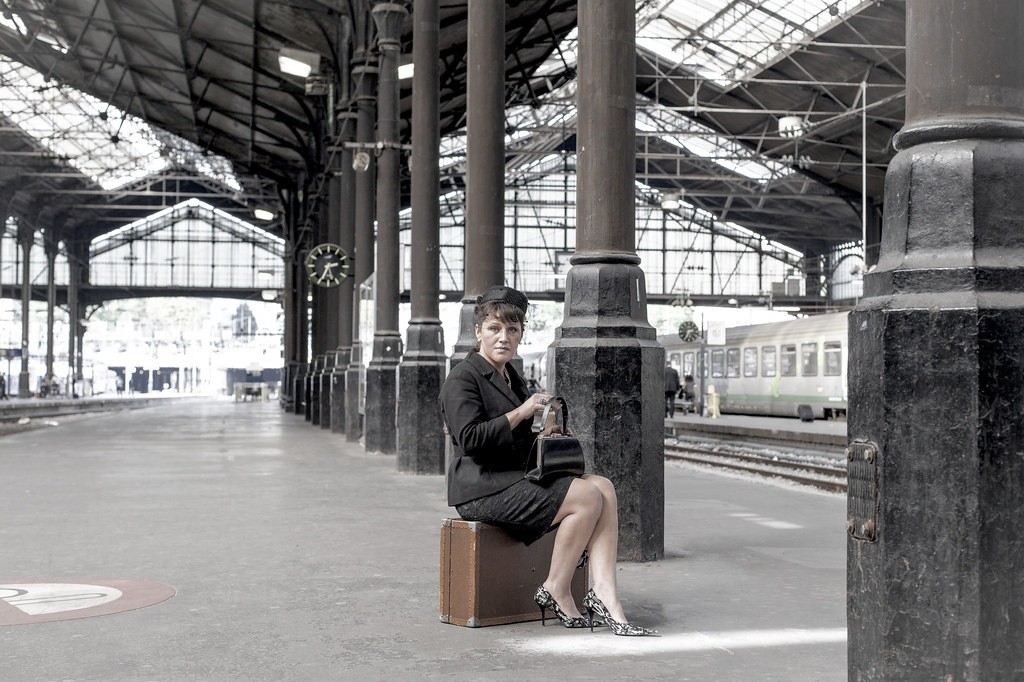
[477,286,528,314]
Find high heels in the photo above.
[534,585,603,628]
[582,588,658,635]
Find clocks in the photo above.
[305,243,350,287]
[679,321,700,342]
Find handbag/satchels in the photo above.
[524,396,584,482]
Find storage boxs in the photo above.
[439,517,589,627]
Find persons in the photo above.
[683,375,697,414]
[665,360,679,418]
[437,285,662,637]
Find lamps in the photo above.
[661,189,681,209]
[255,205,284,221]
[278,48,336,78]
[778,112,803,137]
[262,290,285,300]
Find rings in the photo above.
[539,397,546,405]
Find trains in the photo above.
[657,309,853,422]
